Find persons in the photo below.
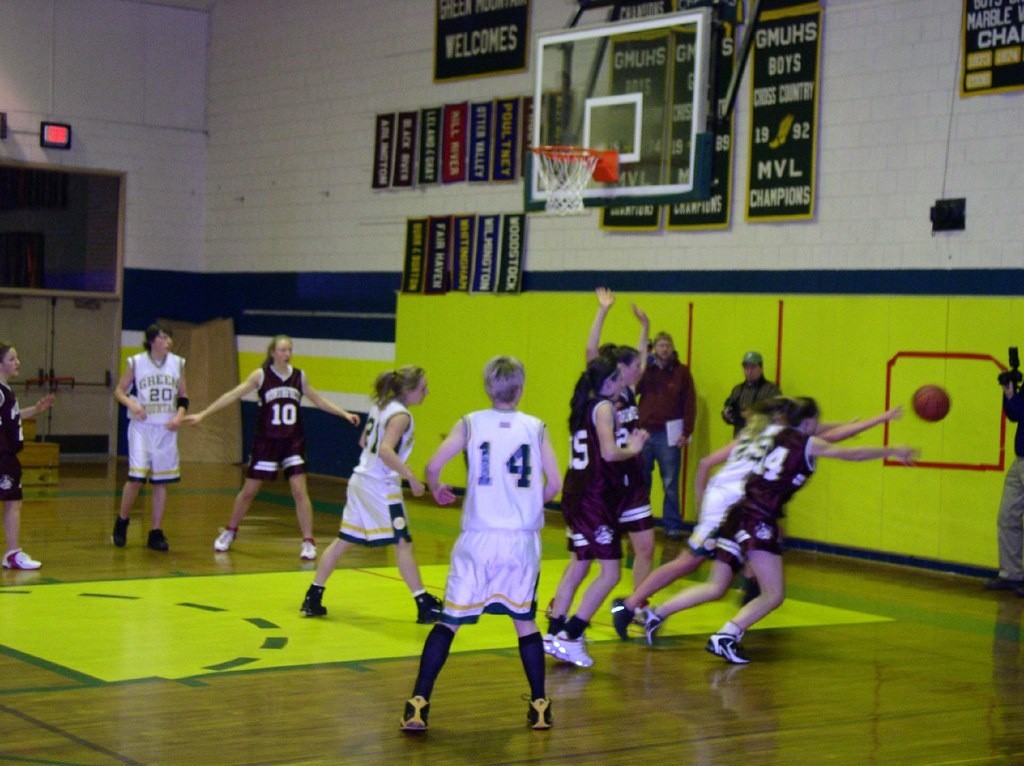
[631,331,696,536]
[612,398,920,664]
[298,365,444,626]
[722,351,781,435]
[541,287,655,667]
[183,334,360,560]
[398,355,553,732]
[0,341,54,571]
[982,381,1024,591]
[113,322,189,552]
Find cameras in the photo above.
[998,347,1022,391]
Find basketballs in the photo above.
[912,384,951,422]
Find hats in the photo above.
[742,352,761,365]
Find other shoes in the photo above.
[666,528,681,540]
[981,573,1021,590]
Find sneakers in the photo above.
[639,608,662,647]
[705,632,751,664]
[522,692,553,729]
[148,530,169,551]
[301,539,316,559]
[215,526,236,552]
[302,589,327,615]
[2,549,42,570]
[612,597,633,640]
[414,597,443,624]
[542,630,594,667]
[113,516,130,546]
[400,696,430,730]
